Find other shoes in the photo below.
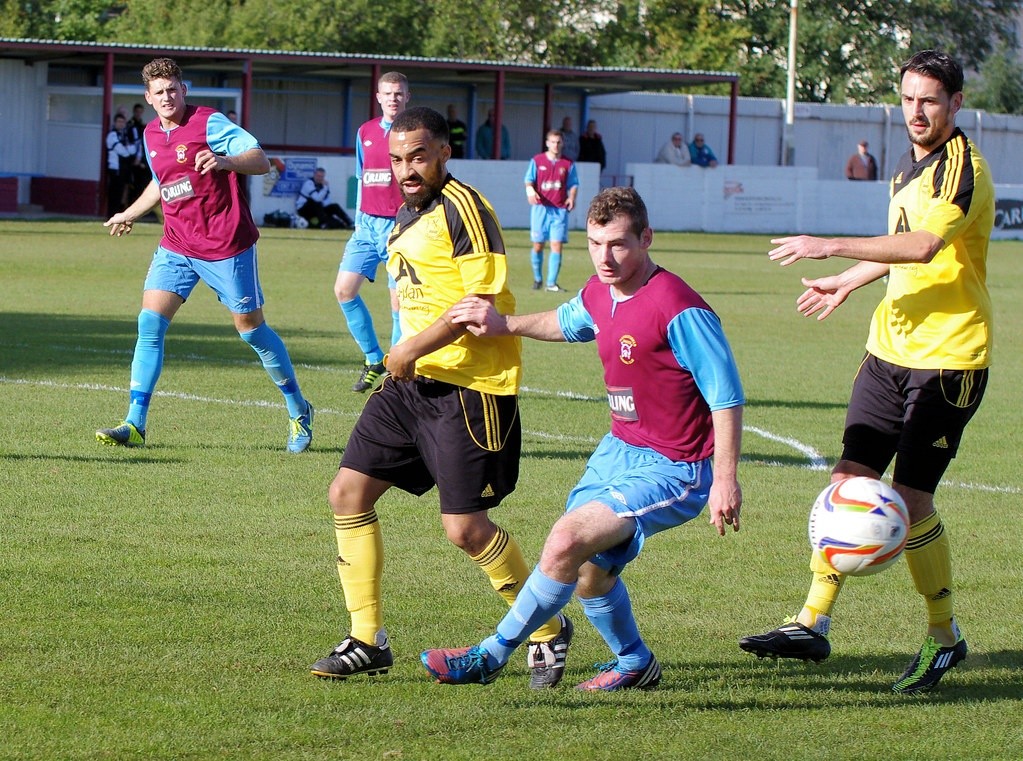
[546,284,565,292]
[534,281,542,292]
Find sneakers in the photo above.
[421,647,506,685]
[96,421,145,449]
[574,647,661,693]
[526,615,575,691]
[352,359,385,392]
[739,613,835,668]
[287,399,313,453]
[308,630,394,680]
[893,617,967,694]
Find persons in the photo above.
[310,106,573,690]
[334,72,410,393]
[559,117,580,161]
[446,105,468,159]
[524,130,579,293]
[577,120,606,170]
[476,109,511,160]
[687,133,718,166]
[419,186,746,692]
[296,168,355,229]
[845,141,877,180]
[106,104,153,222]
[95,58,315,454]
[738,50,995,695]
[226,110,237,122]
[655,133,691,167]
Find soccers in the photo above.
[809,475,912,577]
